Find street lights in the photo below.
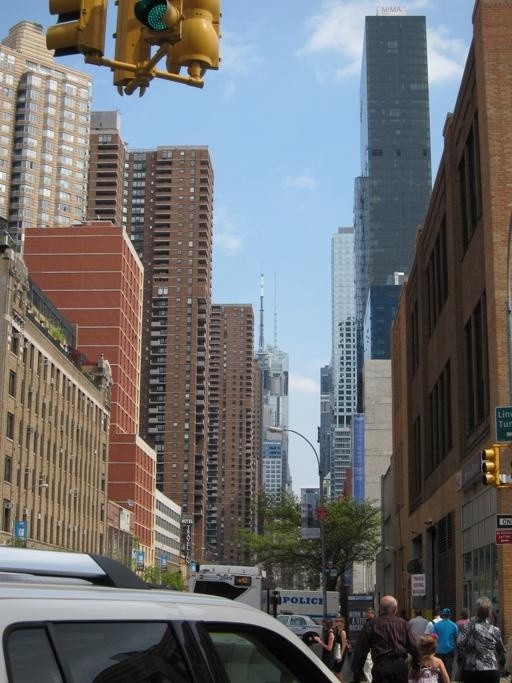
[269,427,327,619]
[16,484,48,547]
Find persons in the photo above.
[314,595,507,683]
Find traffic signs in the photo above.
[495,406,512,443]
[497,513,512,528]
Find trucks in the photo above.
[276,590,339,618]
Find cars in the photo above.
[271,615,323,646]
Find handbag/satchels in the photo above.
[334,644,342,662]
[454,657,464,681]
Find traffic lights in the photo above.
[46,0,222,70]
[482,448,500,486]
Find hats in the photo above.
[441,608,452,615]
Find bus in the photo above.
[189,565,281,618]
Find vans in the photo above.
[0,547,342,683]
